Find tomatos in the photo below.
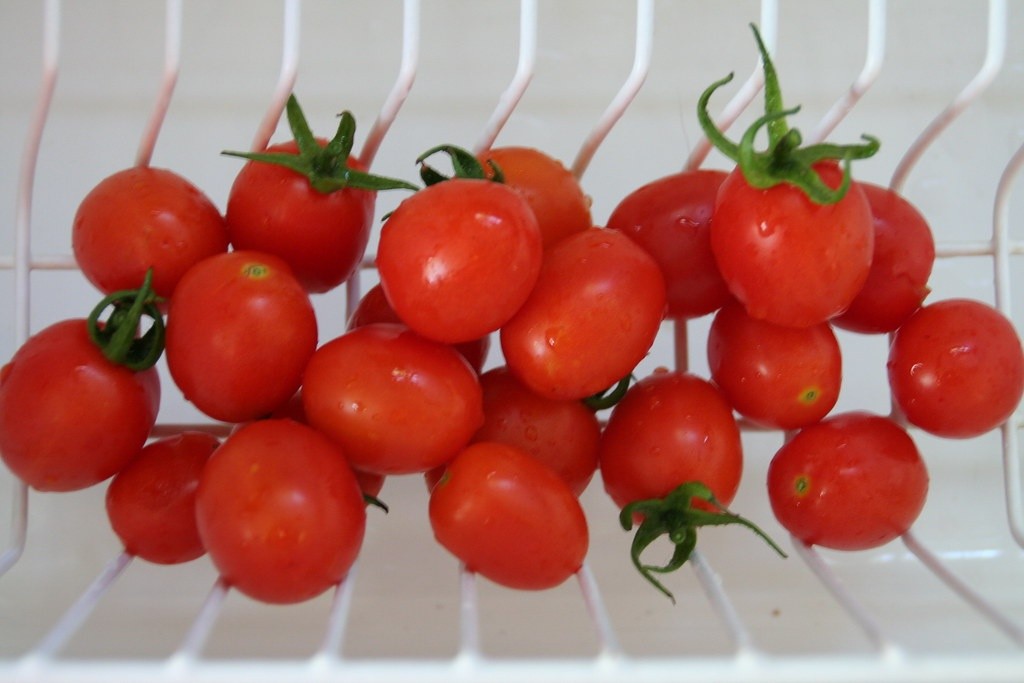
[1,22,1024,603]
